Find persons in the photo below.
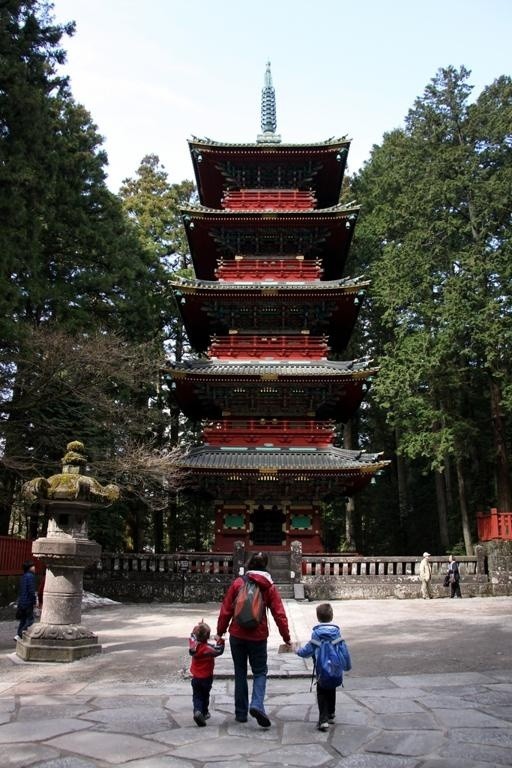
[13,560,37,641]
[446,554,461,598]
[418,551,436,600]
[38,569,46,609]
[188,617,225,725]
[213,552,294,727]
[287,603,352,729]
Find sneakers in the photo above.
[191,708,337,733]
[422,594,465,601]
[13,635,23,642]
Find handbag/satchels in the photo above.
[443,576,458,587]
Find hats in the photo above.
[423,552,455,560]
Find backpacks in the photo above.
[310,637,347,690]
[234,574,268,627]
